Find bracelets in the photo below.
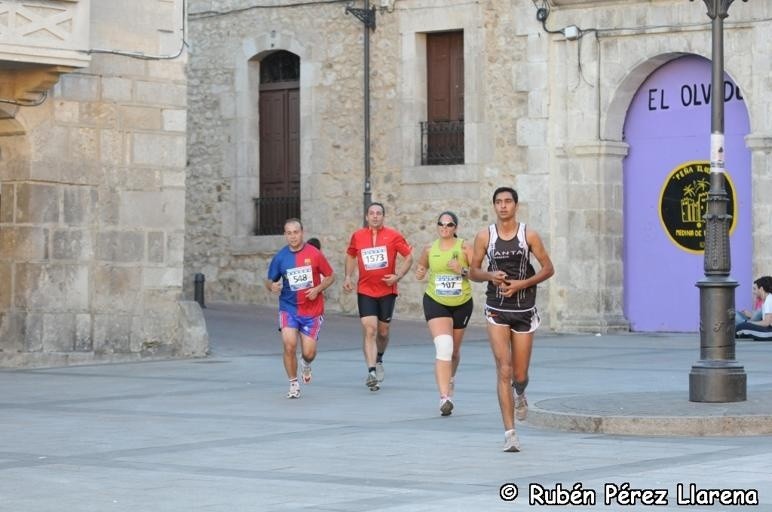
[395,273,400,283]
[461,267,468,276]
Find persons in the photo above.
[469,187,554,451]
[307,238,328,304]
[264,218,335,398]
[415,211,475,415]
[738,280,763,322]
[343,203,413,391]
[733,276,772,341]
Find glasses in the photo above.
[438,221,456,227]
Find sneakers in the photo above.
[302,367,312,384]
[503,433,520,453]
[287,383,300,398]
[440,399,455,416]
[376,363,384,383]
[366,373,380,391]
[514,388,528,420]
